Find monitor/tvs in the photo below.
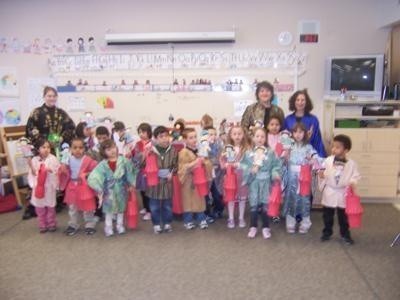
[325,53,385,102]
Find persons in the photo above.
[219,115,319,241]
[240,82,326,225]
[63,113,225,236]
[27,138,60,233]
[319,135,361,245]
[22,86,75,220]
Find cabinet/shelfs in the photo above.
[0,127,30,209]
[322,96,400,205]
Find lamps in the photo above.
[105,31,236,45]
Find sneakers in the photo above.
[139,208,152,222]
[23,206,31,220]
[227,217,271,239]
[38,224,125,236]
[342,236,354,245]
[285,215,312,235]
[321,234,330,240]
[150,211,215,234]
